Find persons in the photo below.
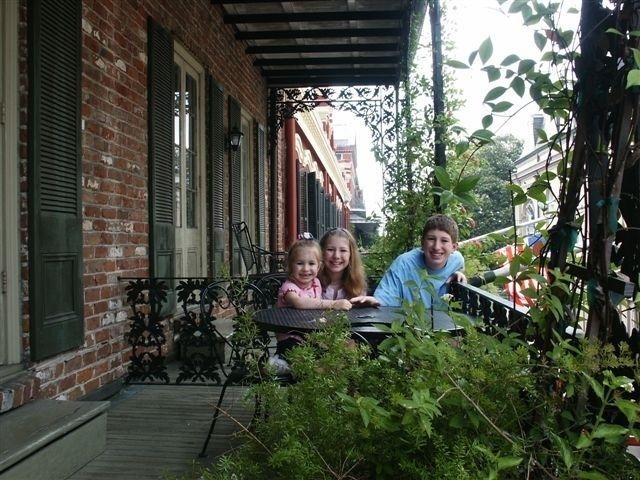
[274,238,352,375]
[373,214,467,349]
[317,227,380,350]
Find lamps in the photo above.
[222,121,243,153]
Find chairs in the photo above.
[234,220,291,278]
[198,278,294,462]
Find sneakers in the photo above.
[263,354,291,375]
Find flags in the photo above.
[490,232,551,309]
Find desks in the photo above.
[251,304,479,356]
